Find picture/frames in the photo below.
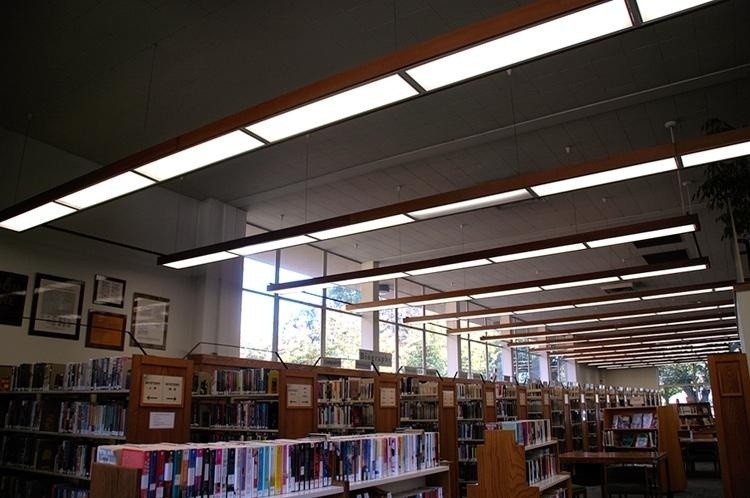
[716,360,744,397]
[1,271,172,351]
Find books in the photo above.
[603,413,658,448]
[677,405,712,431]
[526,382,545,420]
[317,375,376,436]
[0,357,132,498]
[494,382,518,422]
[454,383,486,460]
[131,431,444,497]
[525,450,566,498]
[485,419,552,443]
[398,377,440,432]
[549,386,606,448]
[191,366,281,442]
